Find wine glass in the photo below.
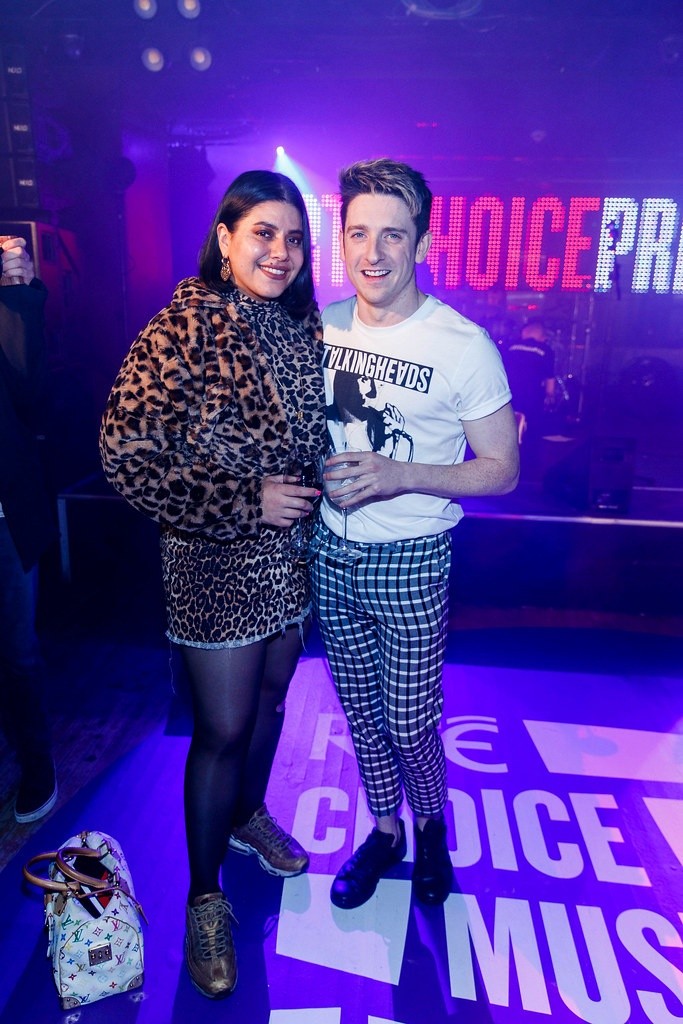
[324,447,364,559]
[282,450,322,559]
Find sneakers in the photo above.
[15,748,58,823]
[228,803,310,877]
[186,893,239,999]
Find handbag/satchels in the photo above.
[23,831,149,1009]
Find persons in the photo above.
[98,169,327,999]
[0,233,62,823]
[478,283,558,435]
[309,158,521,910]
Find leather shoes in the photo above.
[330,818,407,909]
[412,815,454,905]
